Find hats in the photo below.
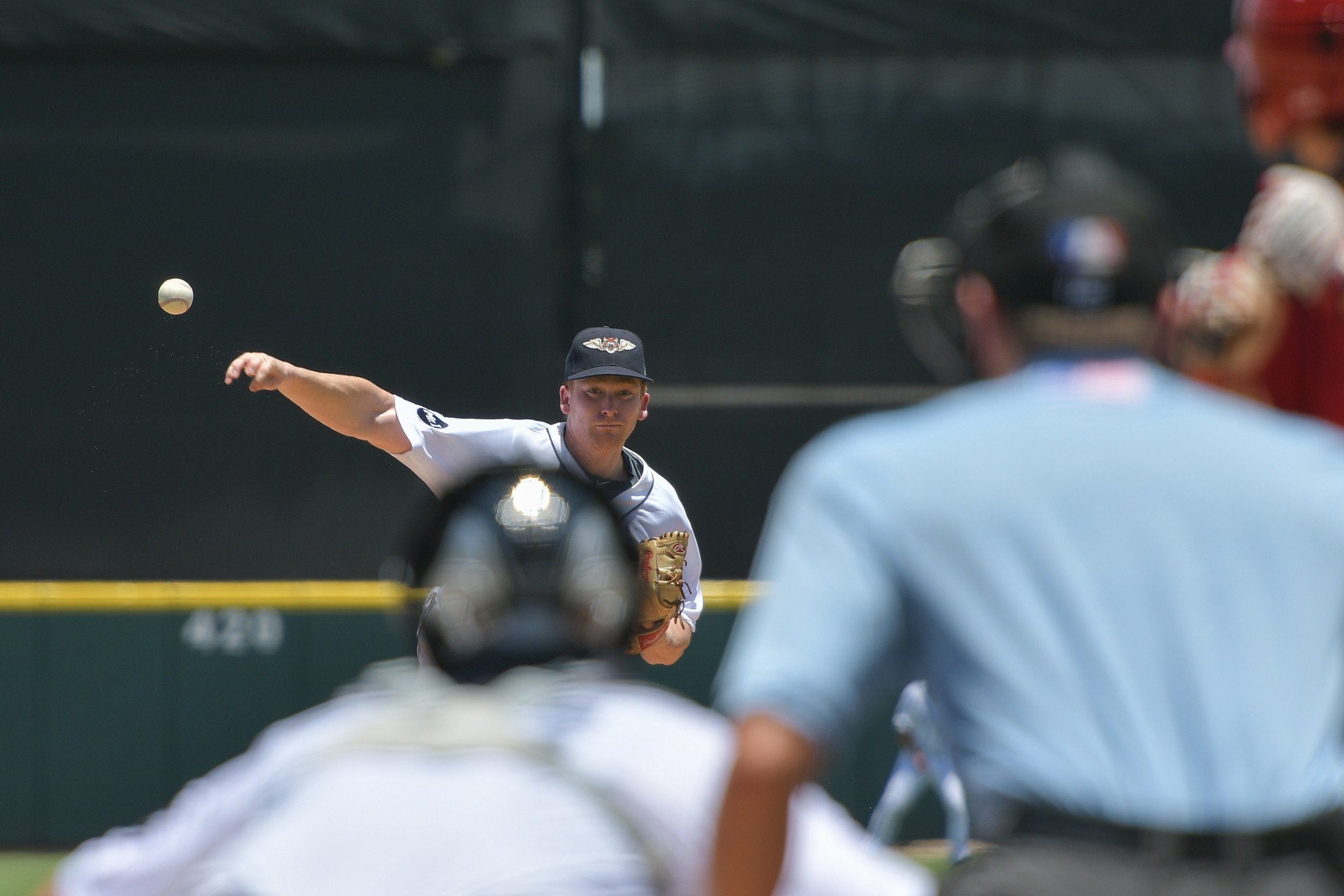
[564,324,652,384]
[969,146,1160,307]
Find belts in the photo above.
[965,792,1342,859]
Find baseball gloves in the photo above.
[623,530,691,657]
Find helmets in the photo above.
[1228,0,1344,145]
[415,466,640,683]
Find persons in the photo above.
[224,326,702,668]
[46,467,945,896]
[713,0,1344,896]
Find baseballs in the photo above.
[158,278,194,315]
